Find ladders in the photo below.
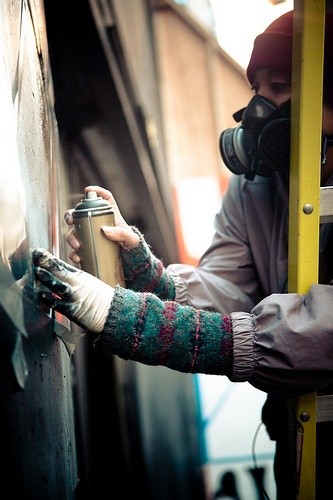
[284,0,333,500]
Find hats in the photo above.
[245,11,333,90]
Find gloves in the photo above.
[33,249,117,334]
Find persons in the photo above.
[31,10,333,500]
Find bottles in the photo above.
[73,192,128,291]
